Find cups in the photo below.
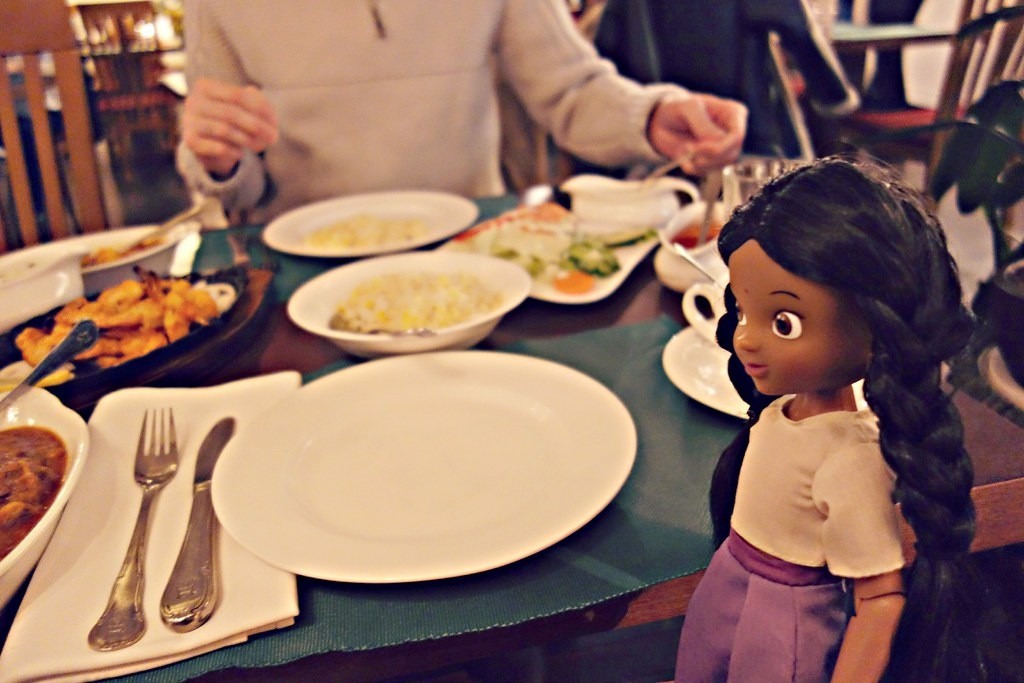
[653,198,732,297]
[685,273,729,343]
[562,172,699,232]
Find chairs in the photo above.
[835,2,1023,203]
[0,0,109,252]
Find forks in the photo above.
[89,401,178,655]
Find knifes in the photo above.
[160,416,236,632]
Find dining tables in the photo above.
[1,186,1023,683]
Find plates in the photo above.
[427,213,661,305]
[262,186,479,261]
[210,351,637,589]
[1,263,250,399]
[661,318,869,424]
[0,386,89,608]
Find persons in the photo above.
[177,0,750,223]
[676,162,979,683]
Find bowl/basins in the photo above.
[0,222,192,294]
[288,252,528,357]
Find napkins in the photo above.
[2,362,300,681]
